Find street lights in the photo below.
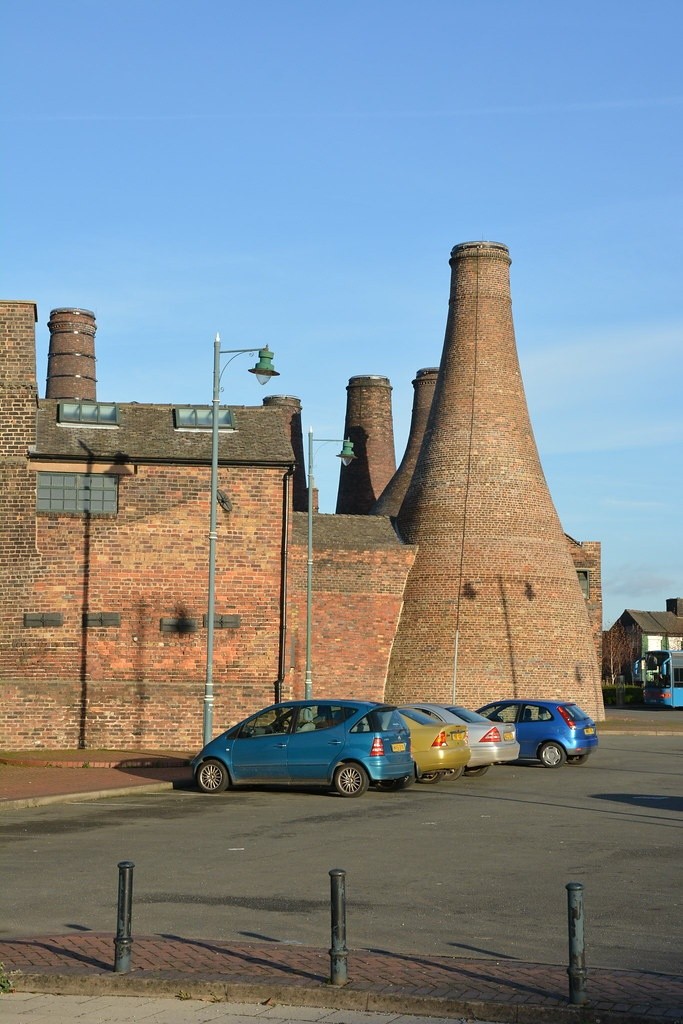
[204,346,280,747]
[306,439,357,718]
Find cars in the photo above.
[310,705,471,789]
[189,701,416,798]
[474,700,599,769]
[401,705,520,777]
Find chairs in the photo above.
[299,708,315,733]
[523,709,534,721]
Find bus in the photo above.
[632,650,683,709]
